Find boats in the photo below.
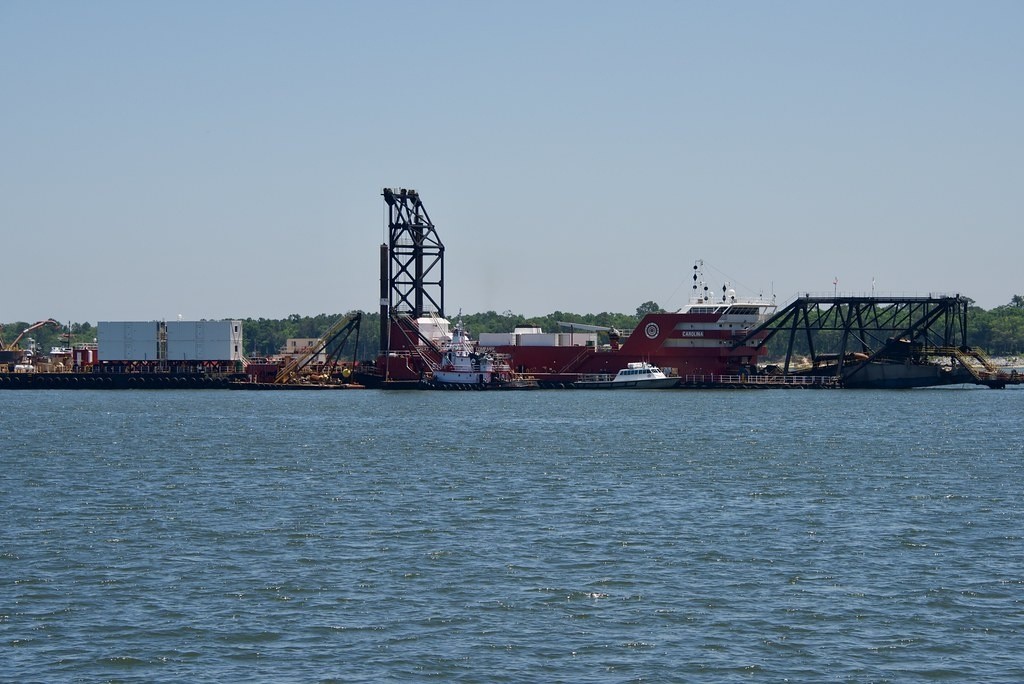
[428,306,496,391]
[572,361,684,389]
